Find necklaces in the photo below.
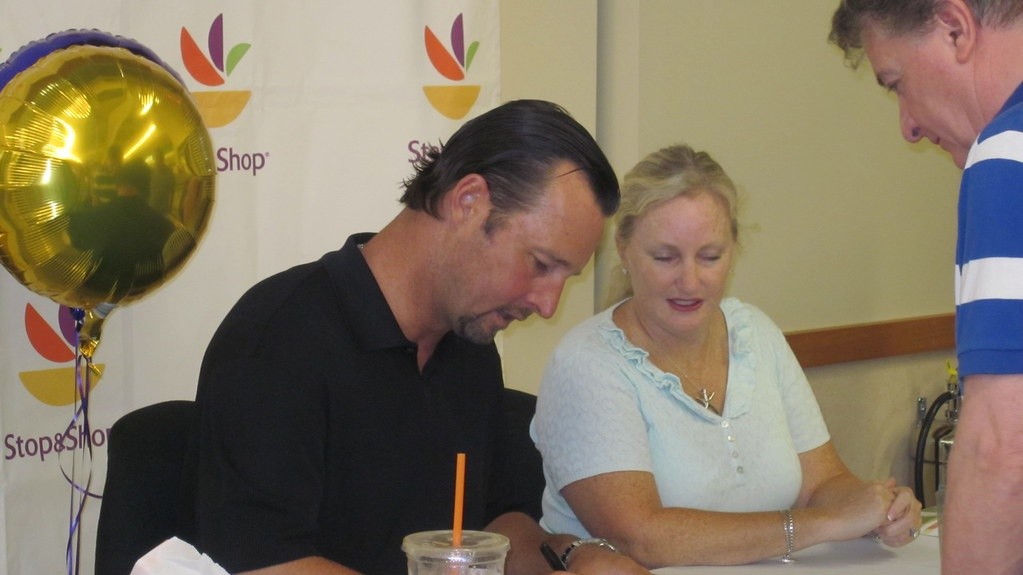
[633,297,720,417]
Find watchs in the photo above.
[561,537,620,569]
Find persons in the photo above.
[151,147,176,212]
[67,158,174,300]
[529,144,923,567]
[826,0,1023,574]
[90,144,132,208]
[195,98,657,574]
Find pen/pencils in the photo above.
[539,542,567,572]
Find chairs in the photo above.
[504,388,546,523]
[93,400,196,575]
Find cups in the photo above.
[402,530,509,575]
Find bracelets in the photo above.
[779,508,795,562]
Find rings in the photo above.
[910,527,920,539]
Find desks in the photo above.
[654,527,940,575]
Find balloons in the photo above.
[0,26,217,313]
